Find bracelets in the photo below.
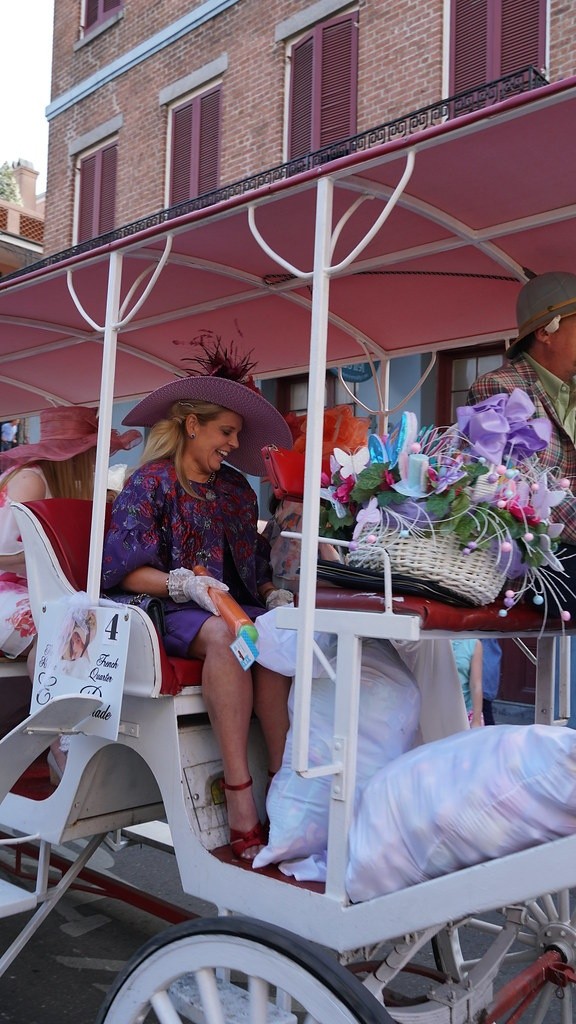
[166,577,171,595]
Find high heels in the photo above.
[220,775,268,863]
[47,750,63,785]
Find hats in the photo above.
[50,608,98,656]
[503,271,576,359]
[121,328,293,477]
[0,406,144,474]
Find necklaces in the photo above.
[207,472,216,481]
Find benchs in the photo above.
[12,495,268,855]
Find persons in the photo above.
[463,271,576,546]
[0,405,143,776]
[261,481,501,728]
[102,341,294,862]
[1,418,21,452]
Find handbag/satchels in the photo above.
[261,446,332,505]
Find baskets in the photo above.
[346,422,537,606]
